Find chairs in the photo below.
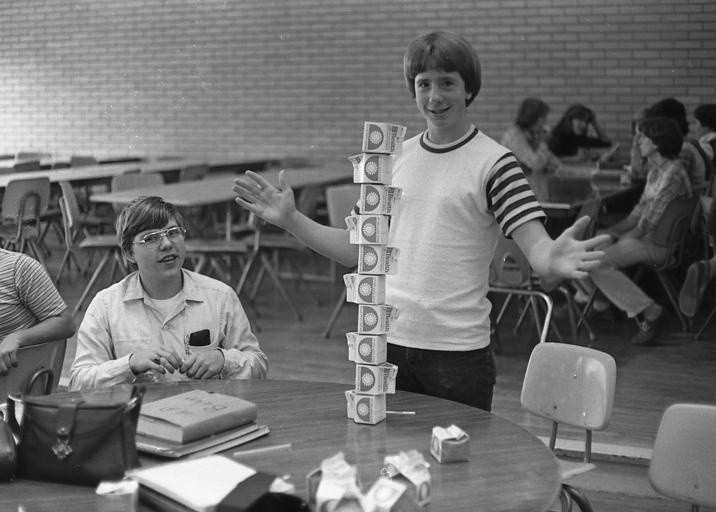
[3,147,715,371]
[642,400,716,511]
[513,340,621,511]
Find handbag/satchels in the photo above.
[6,364,146,490]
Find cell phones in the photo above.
[189,328,210,346]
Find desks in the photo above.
[2,373,564,510]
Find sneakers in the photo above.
[631,306,672,346]
[551,298,626,324]
[677,259,711,319]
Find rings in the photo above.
[153,355,161,365]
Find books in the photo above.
[132,388,257,454]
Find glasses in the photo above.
[126,224,187,250]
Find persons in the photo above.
[0,246,76,375]
[232,29,611,414]
[575,94,715,346]
[545,104,612,160]
[68,195,270,392]
[502,95,565,174]
